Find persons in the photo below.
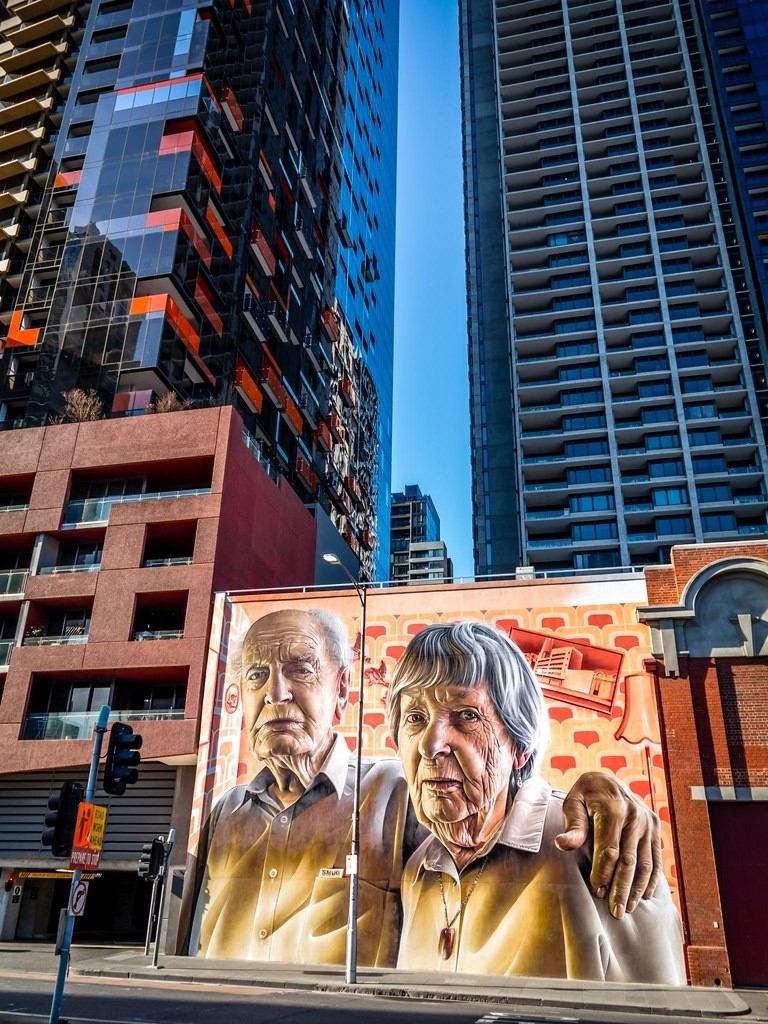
[384,621,688,987]
[171,608,662,969]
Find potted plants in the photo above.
[75,627,85,635]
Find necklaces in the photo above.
[437,856,488,962]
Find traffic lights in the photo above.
[139,838,164,879]
[42,781,85,857]
[103,721,143,796]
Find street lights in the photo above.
[324,552,369,984]
